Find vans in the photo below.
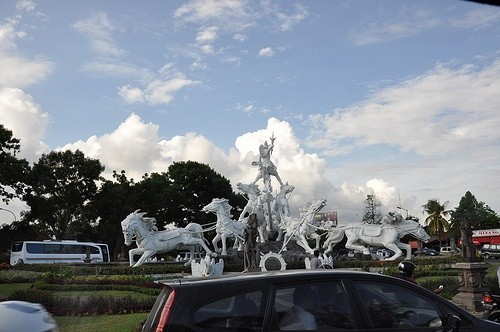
[180,252,202,264]
[479,244,500,258]
[438,246,461,256]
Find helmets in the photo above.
[398,260,417,278]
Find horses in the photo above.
[323,211,430,261]
[201,198,248,256]
[272,181,295,242]
[237,182,269,243]
[278,198,327,255]
[121,209,218,268]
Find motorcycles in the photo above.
[480,291,500,323]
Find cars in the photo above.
[414,246,438,256]
[141,269,500,332]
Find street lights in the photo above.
[397,207,411,242]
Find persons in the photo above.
[354,288,390,329]
[311,250,323,269]
[318,282,339,303]
[279,285,317,330]
[396,261,441,294]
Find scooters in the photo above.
[425,284,444,311]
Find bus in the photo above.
[10,240,110,267]
